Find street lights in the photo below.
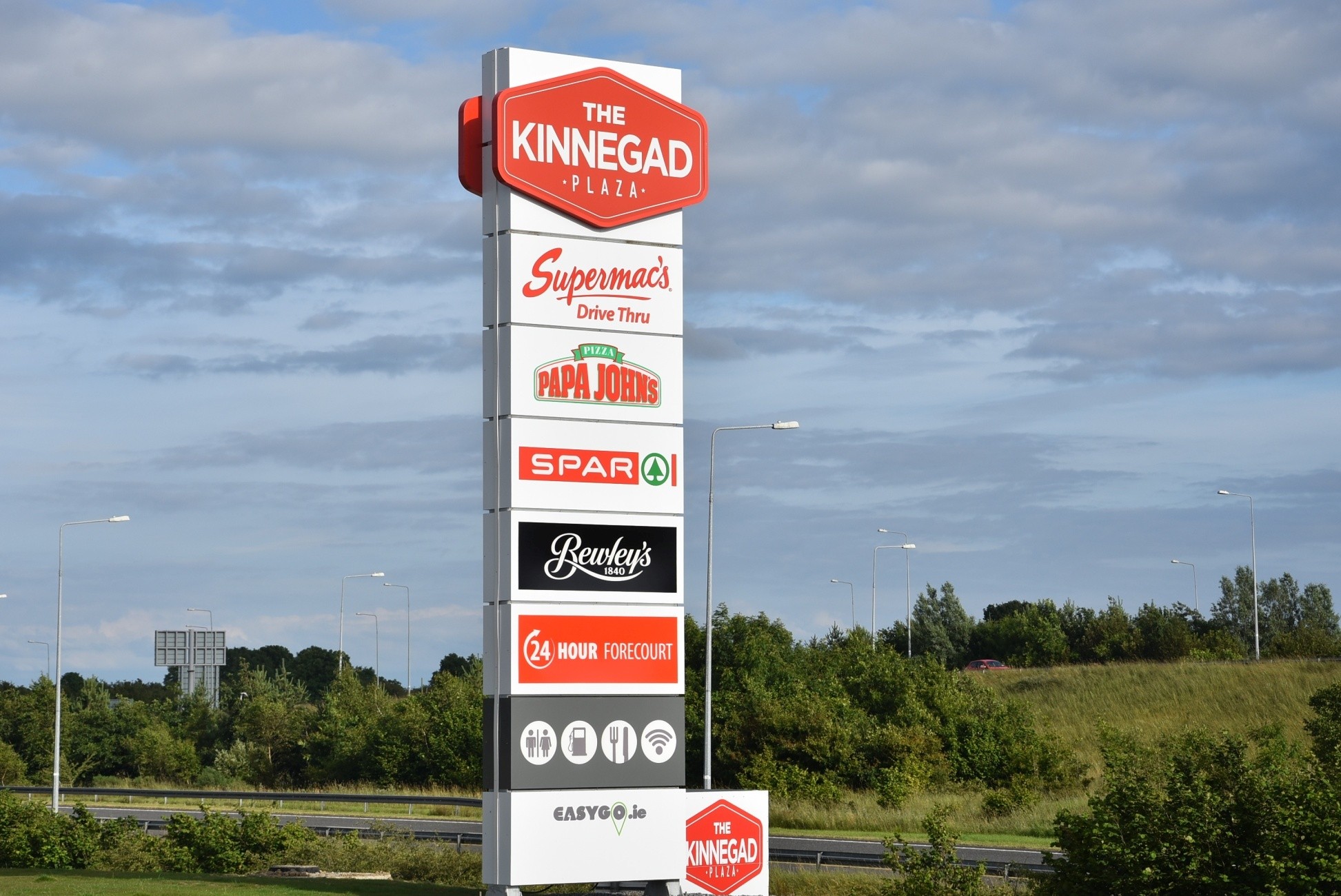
[52,514,130,807]
[709,419,800,793]
[338,573,412,701]
[184,608,213,632]
[28,640,49,683]
[1218,489,1260,662]
[1171,558,1199,617]
[830,578,854,638]
[871,529,916,659]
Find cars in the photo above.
[967,659,1008,671]
